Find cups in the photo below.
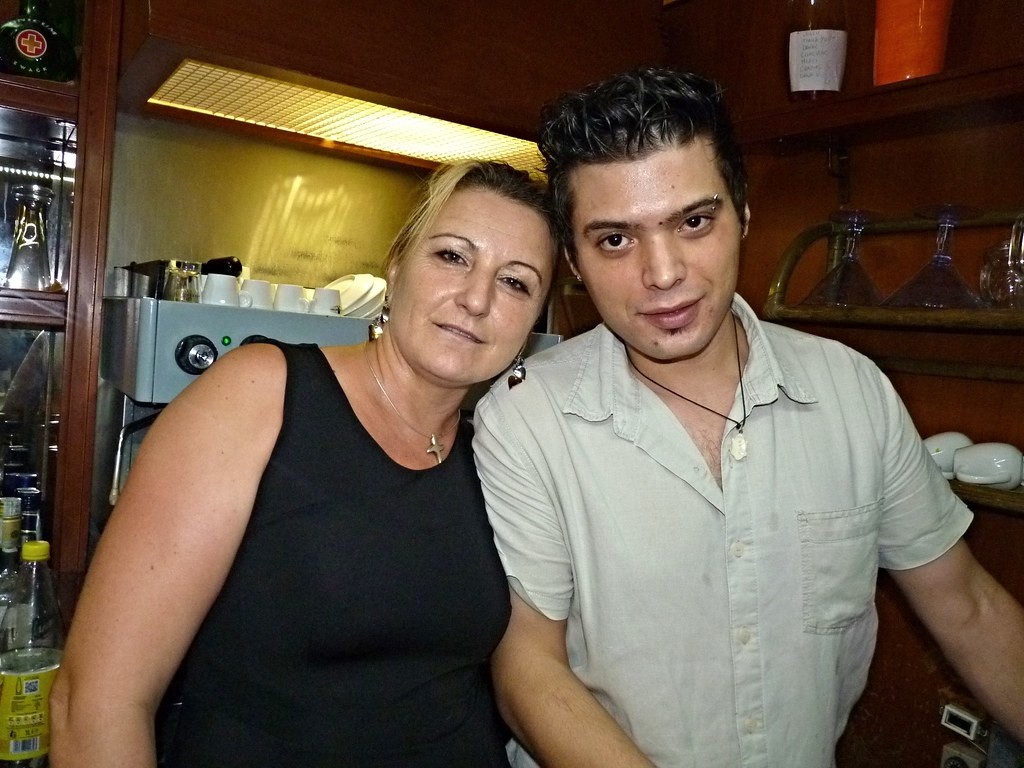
[163,260,200,303]
[954,442,1023,490]
[202,273,238,307]
[274,284,307,315]
[4,185,56,292]
[310,288,340,318]
[241,280,273,311]
[924,432,972,480]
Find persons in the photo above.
[48,167,559,768]
[475,71,1024,768]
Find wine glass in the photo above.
[800,209,885,307]
[878,202,984,309]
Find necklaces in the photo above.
[362,340,461,465]
[628,311,752,459]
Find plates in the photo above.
[322,274,387,320]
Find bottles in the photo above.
[980,240,1024,313]
[0,542,22,623]
[0,541,65,768]
[784,0,848,104]
[15,488,42,547]
[0,497,22,542]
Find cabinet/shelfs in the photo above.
[758,208,1023,518]
[0,0,121,631]
